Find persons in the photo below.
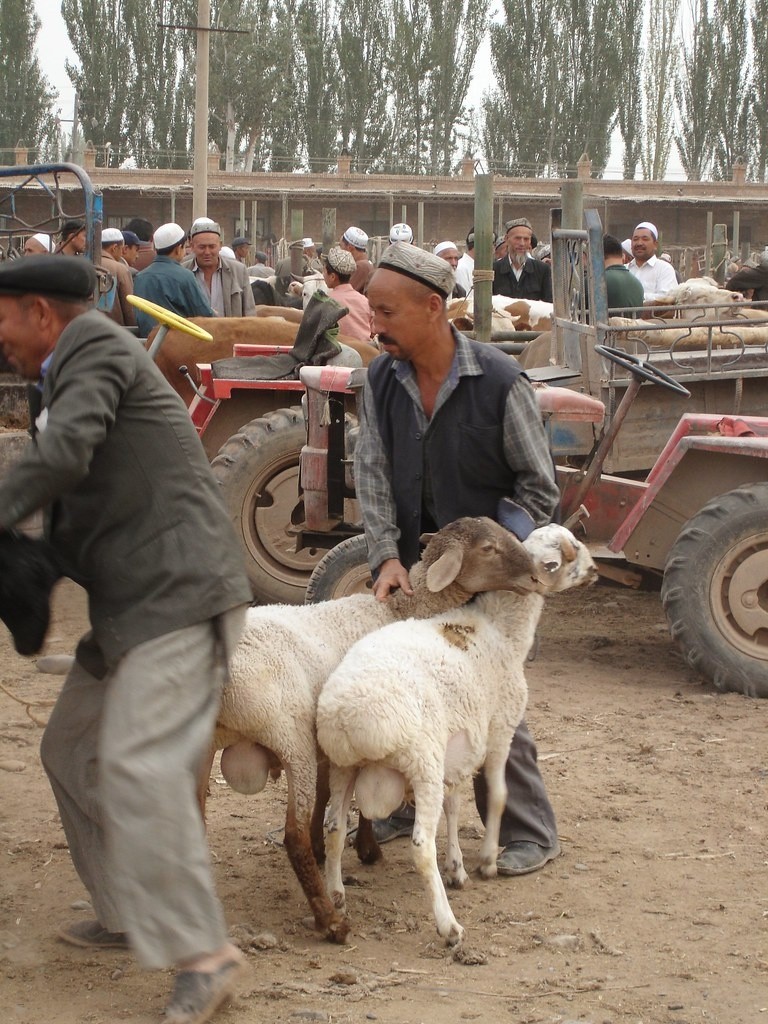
[179,222,257,318]
[493,217,553,303]
[23,216,679,331]
[0,254,252,1024]
[580,233,644,325]
[724,250,768,311]
[94,228,137,327]
[322,249,378,347]
[353,241,561,875]
[131,223,217,338]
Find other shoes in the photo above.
[345,800,415,845]
[55,918,136,948]
[496,841,561,876]
[160,944,246,1024]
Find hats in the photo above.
[327,248,357,275]
[0,254,98,303]
[468,218,551,259]
[232,237,253,246]
[255,252,267,261]
[153,223,185,249]
[389,223,413,244]
[377,240,458,300]
[662,253,671,263]
[121,231,150,245]
[343,227,369,249]
[301,237,314,248]
[635,221,658,240]
[190,217,220,239]
[32,233,56,253]
[433,241,458,256]
[621,239,634,257]
[102,228,124,242]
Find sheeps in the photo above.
[190,515,541,932]
[315,522,599,943]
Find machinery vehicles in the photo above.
[285,344,768,701]
[1,162,768,604]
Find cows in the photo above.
[146,268,385,466]
[445,274,768,349]
